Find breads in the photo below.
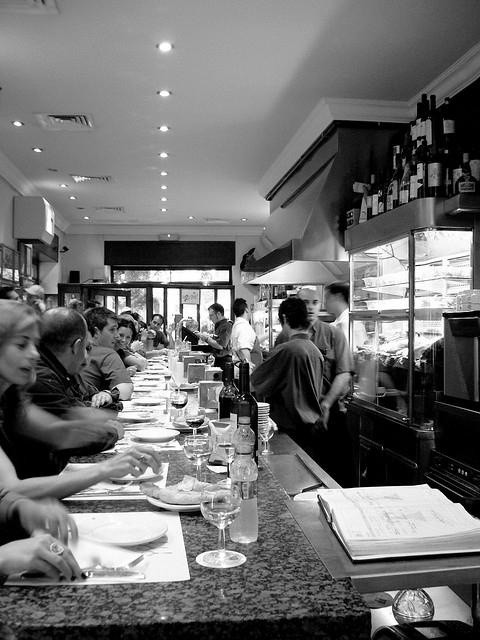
[140,481,221,504]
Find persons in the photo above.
[147,312,215,348]
[274,289,358,491]
[1,487,88,586]
[228,320,234,327]
[322,279,368,369]
[250,296,327,469]
[229,298,265,397]
[193,303,234,384]
[2,298,160,500]
[2,285,169,474]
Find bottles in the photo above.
[232,415,256,460]
[216,358,237,444]
[366,91,479,222]
[232,360,259,465]
[227,444,258,545]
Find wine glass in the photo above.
[184,406,205,439]
[169,391,186,417]
[199,486,243,567]
[165,369,186,393]
[258,423,280,456]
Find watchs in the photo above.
[141,340,145,343]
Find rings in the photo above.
[137,456,145,462]
[50,543,65,555]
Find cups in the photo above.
[183,432,212,484]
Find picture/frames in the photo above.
[0,242,32,289]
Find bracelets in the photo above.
[102,388,122,411]
[205,337,210,344]
[321,400,330,409]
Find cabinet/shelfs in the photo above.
[343,195,477,431]
[359,411,433,488]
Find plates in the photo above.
[254,401,270,440]
[115,358,162,424]
[109,469,164,483]
[75,514,168,547]
[131,428,180,444]
[147,494,201,514]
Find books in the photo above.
[314,482,480,565]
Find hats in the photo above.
[24,285,44,295]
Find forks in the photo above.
[79,555,149,572]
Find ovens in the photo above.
[425,448,479,518]
